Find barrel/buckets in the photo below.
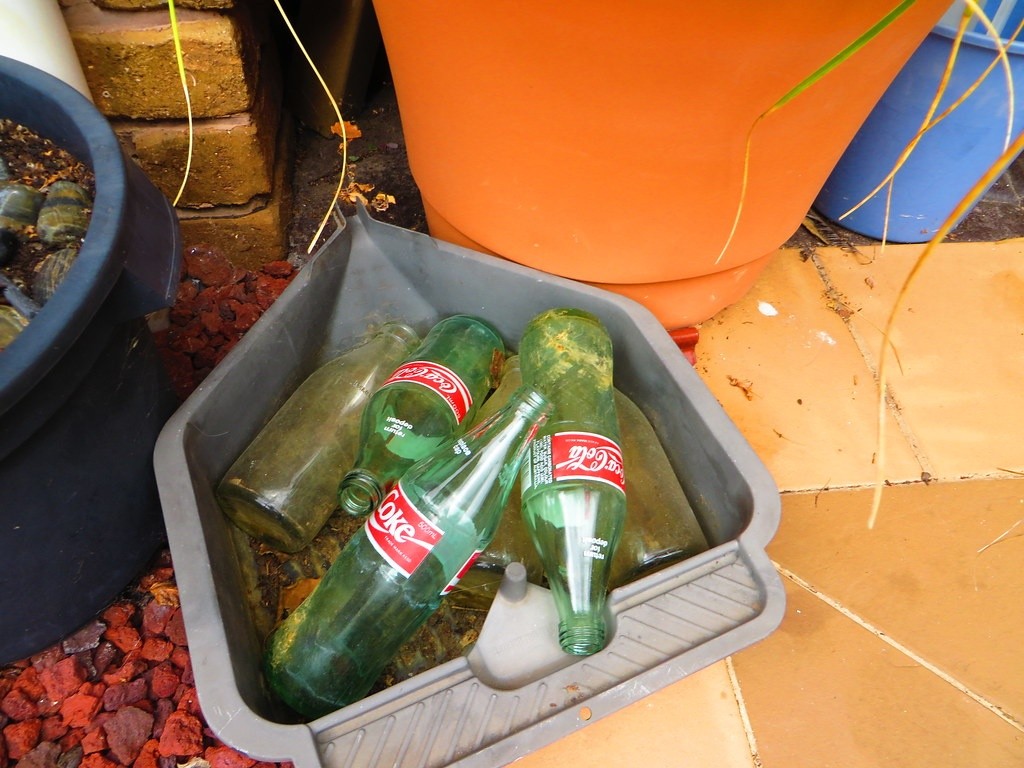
[816,29,1024,241]
[377,2,947,329]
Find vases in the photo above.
[0,56,186,672]
[812,0,1024,249]
[368,0,955,337]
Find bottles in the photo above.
[444,311,708,654]
[336,314,506,518]
[268,385,553,715]
[215,320,421,550]
